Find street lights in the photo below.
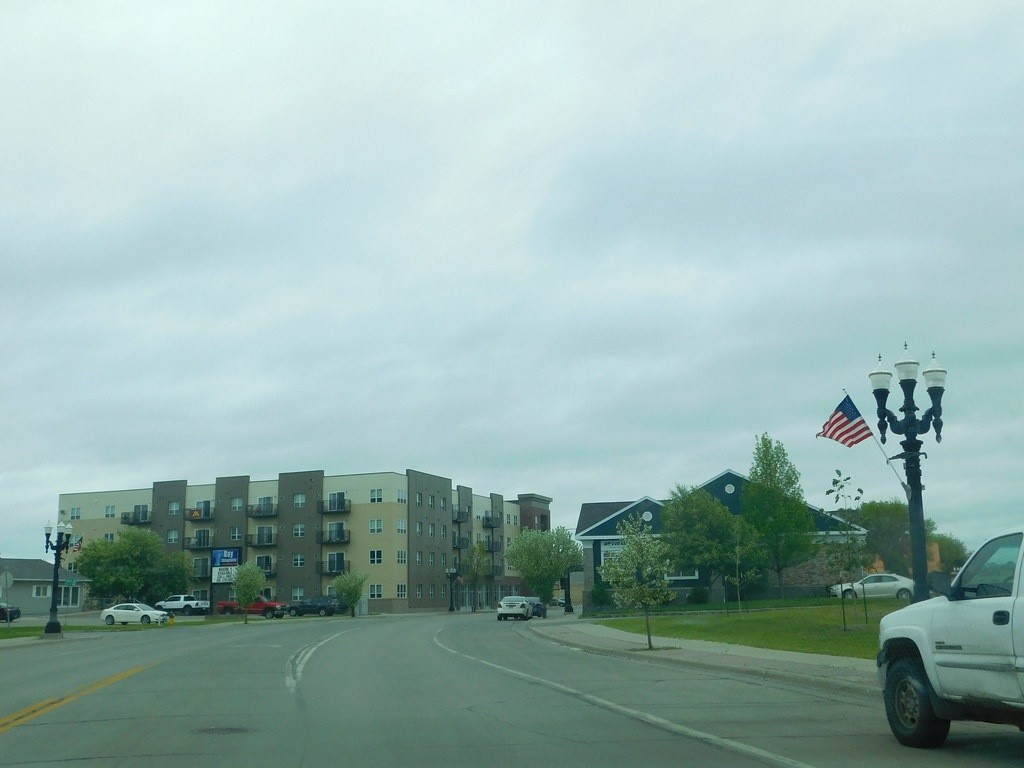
[445,568,457,611]
[867,340,946,605]
[43,519,74,633]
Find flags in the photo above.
[816,394,873,449]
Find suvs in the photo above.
[287,595,349,616]
[876,523,1024,751]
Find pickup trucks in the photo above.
[155,594,210,616]
[218,594,288,618]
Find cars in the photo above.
[549,596,565,607]
[101,602,169,626]
[0,602,21,622]
[827,573,915,601]
[525,596,547,619]
[496,595,534,620]
